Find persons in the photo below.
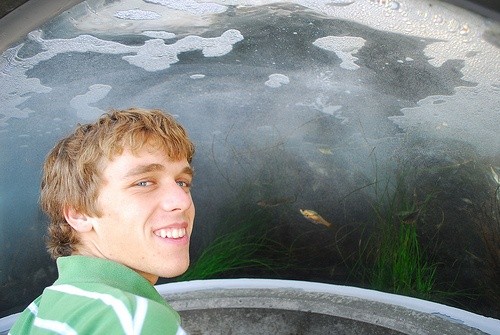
[7,108,196,335]
